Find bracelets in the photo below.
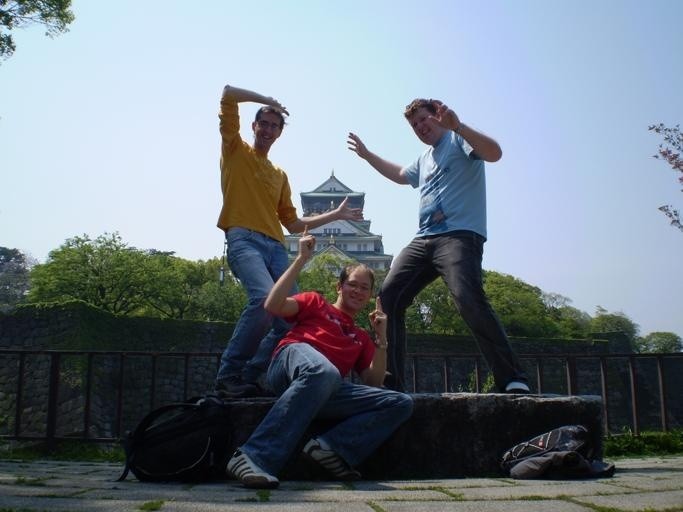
[453,122,465,135]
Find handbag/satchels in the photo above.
[496,424,595,474]
[114,392,234,483]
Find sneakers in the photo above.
[505,380,531,394]
[213,375,259,398]
[224,448,280,489]
[299,437,362,482]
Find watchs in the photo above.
[374,337,388,352]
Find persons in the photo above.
[348,95,533,392]
[208,79,365,396]
[225,219,413,490]
